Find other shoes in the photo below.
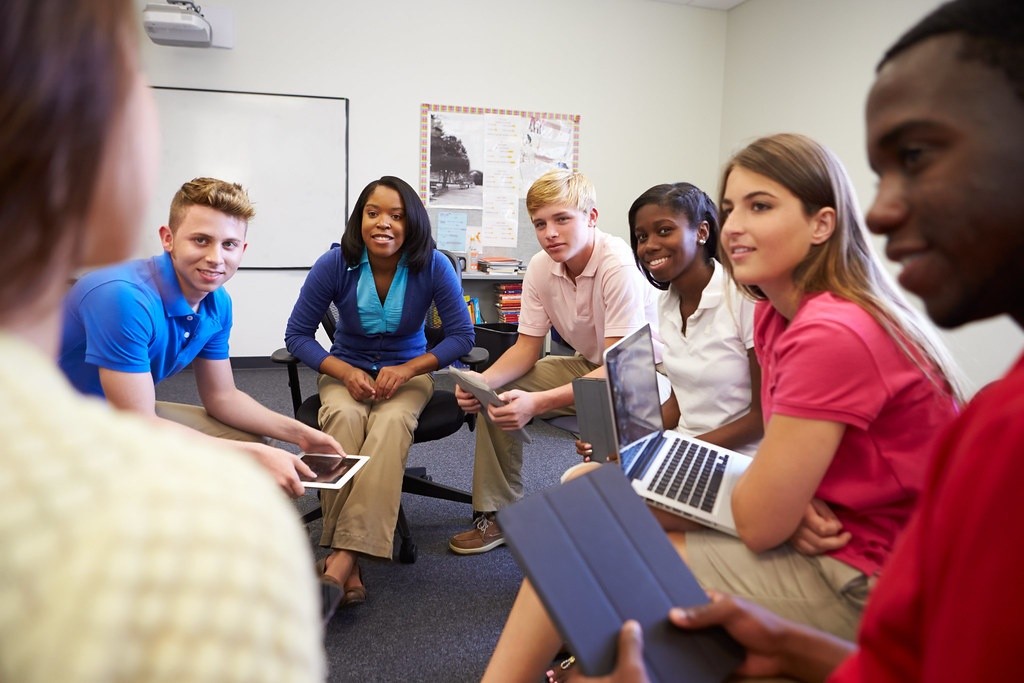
[342,563,366,606]
[314,553,356,596]
[448,512,508,554]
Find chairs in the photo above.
[537,326,582,442]
[272,299,491,566]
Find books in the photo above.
[496,284,523,324]
[433,295,480,327]
[479,255,522,276]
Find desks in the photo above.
[460,271,525,281]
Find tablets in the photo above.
[289,451,370,489]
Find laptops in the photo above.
[601,322,754,538]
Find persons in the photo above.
[449,168,659,557]
[561,0,1024,681]
[629,182,765,459]
[59,178,347,499]
[1,0,330,682]
[478,132,977,683]
[287,176,475,625]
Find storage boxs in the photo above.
[474,320,518,375]
